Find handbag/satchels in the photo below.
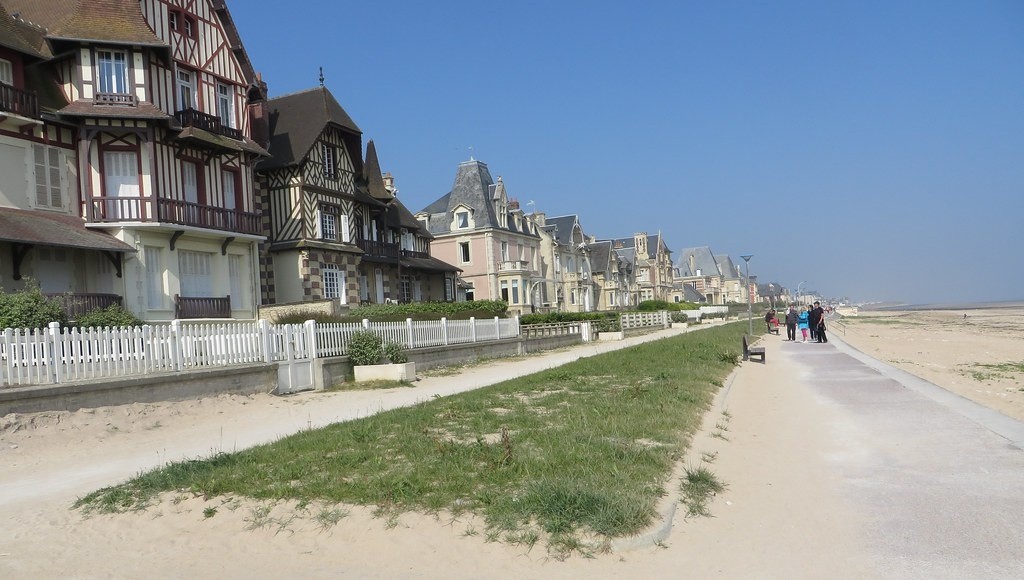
[798,314,808,323]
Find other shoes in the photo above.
[817,341,821,343]
[824,340,827,343]
[801,340,807,343]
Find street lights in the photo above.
[798,279,808,306]
[739,255,754,333]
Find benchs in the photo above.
[743,334,765,364]
[778,317,786,327]
[767,322,778,334]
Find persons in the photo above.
[765,304,817,343]
[813,301,828,343]
